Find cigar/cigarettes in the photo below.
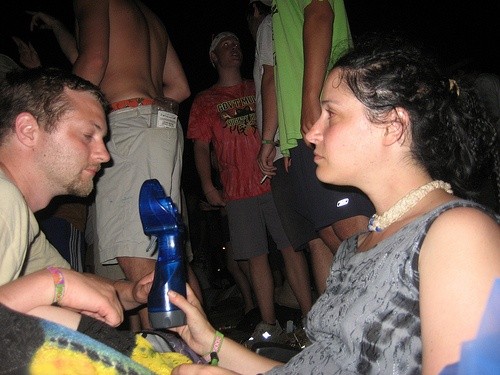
[261,175,268,183]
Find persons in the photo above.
[0,67,155,327]
[186,32,313,345]
[270,0,376,255]
[249,0,334,296]
[0,0,190,332]
[26,41,500,375]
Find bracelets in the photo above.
[262,140,273,144]
[203,330,224,366]
[45,266,65,303]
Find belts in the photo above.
[109,98,158,112]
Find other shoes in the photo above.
[237,309,260,333]
[241,319,288,352]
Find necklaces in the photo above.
[368,180,453,233]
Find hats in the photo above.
[209,32,238,69]
